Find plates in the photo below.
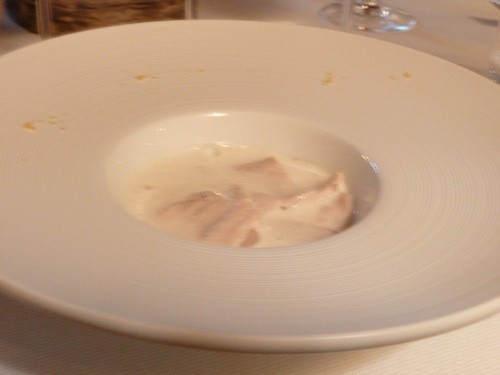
[0,20,500,352]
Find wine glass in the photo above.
[317,0,416,32]
